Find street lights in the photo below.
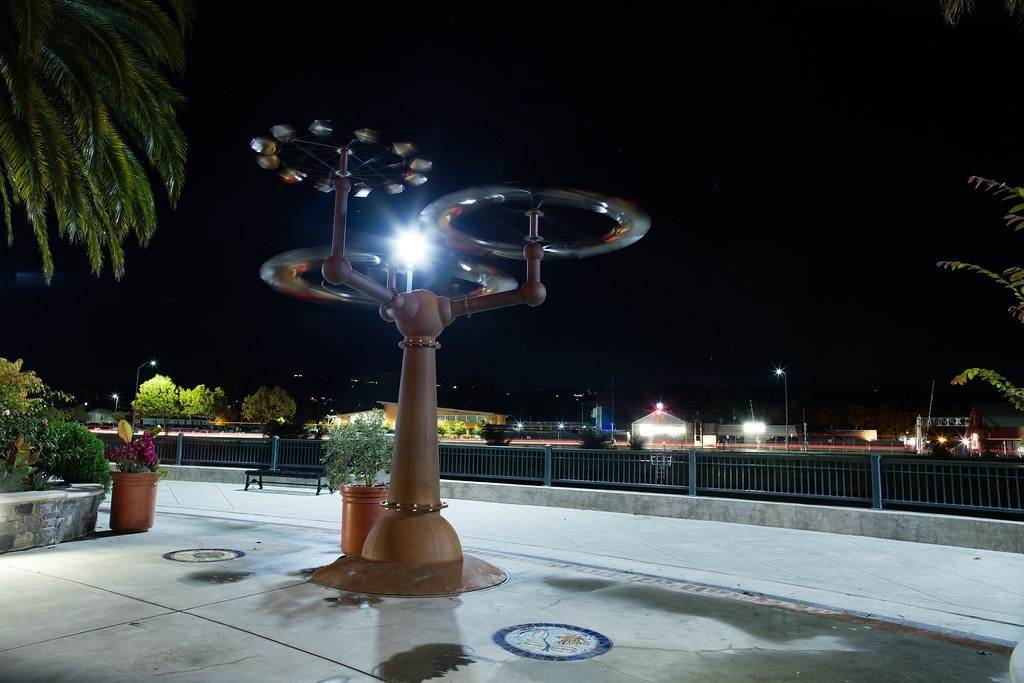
[132,358,159,434]
[112,394,119,426]
[518,423,523,447]
[775,369,789,454]
[558,423,563,448]
[386,226,430,291]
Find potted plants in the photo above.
[326,403,396,561]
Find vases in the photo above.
[109,470,162,534]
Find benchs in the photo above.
[243,469,337,496]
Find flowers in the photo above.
[100,431,161,471]
